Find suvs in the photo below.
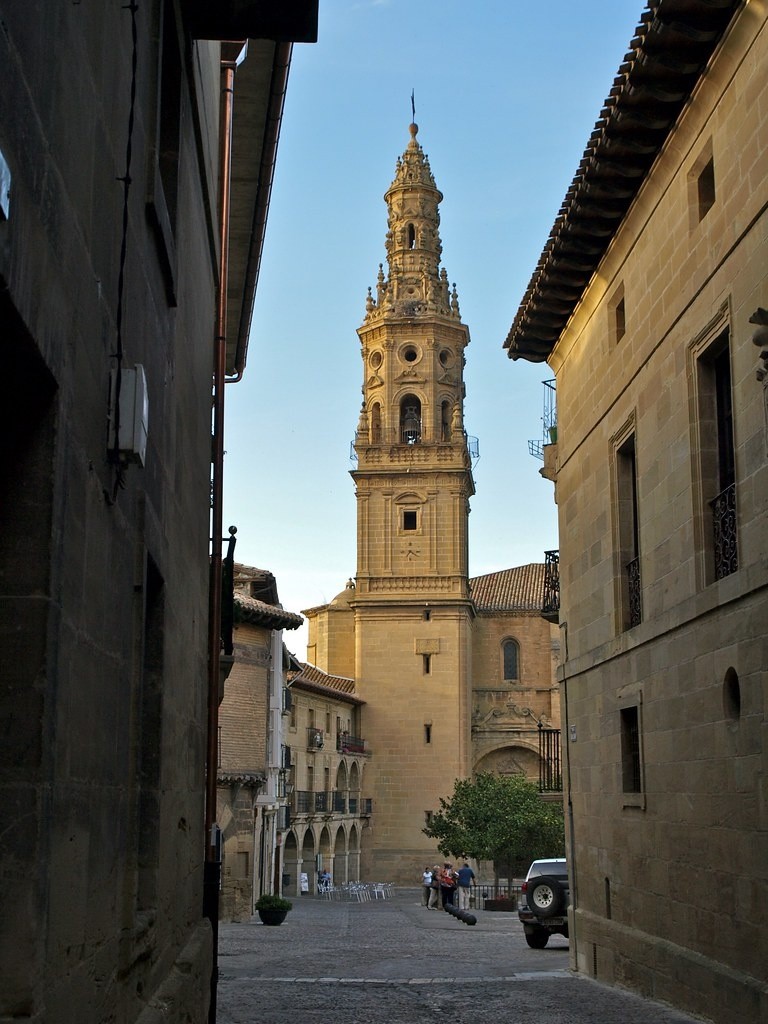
[517,857,568,950]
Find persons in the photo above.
[320,869,332,891]
[421,861,477,911]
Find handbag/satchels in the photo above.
[441,878,455,887]
